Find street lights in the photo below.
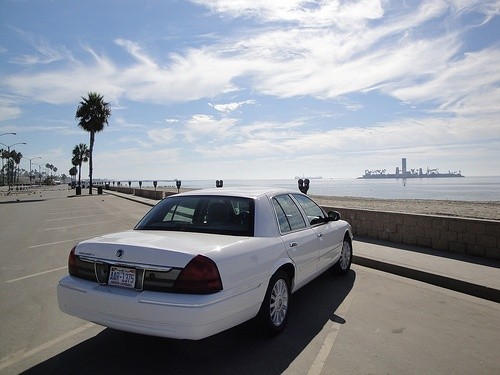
[24,156,41,186]
[1,143,27,191]
[0,133,17,137]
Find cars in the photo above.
[57,187,353,340]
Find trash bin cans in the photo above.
[98,186,102,194]
[106,184,109,190]
[76,187,81,195]
